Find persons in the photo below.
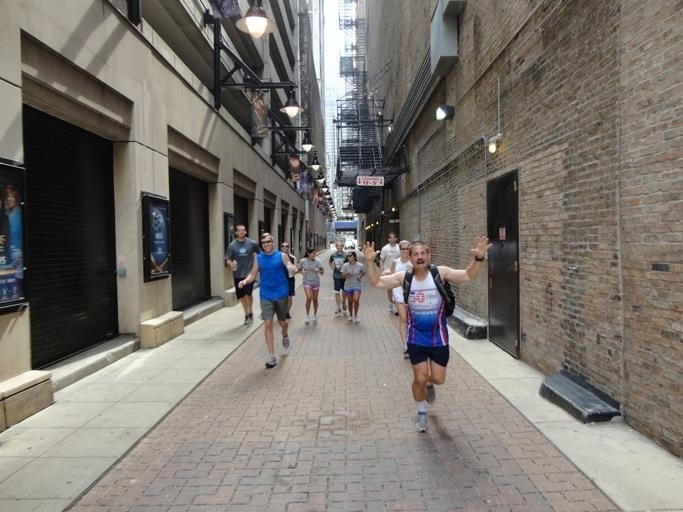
[224,224,262,325]
[340,251,366,323]
[390,240,414,354]
[380,230,401,314]
[237,232,299,367]
[298,247,324,324]
[280,242,298,319]
[4,184,24,250]
[328,241,350,315]
[362,234,493,433]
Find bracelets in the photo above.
[474,255,485,262]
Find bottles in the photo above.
[232,260,238,272]
[288,261,293,278]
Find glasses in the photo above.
[259,237,411,261]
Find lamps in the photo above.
[266,124,315,157]
[219,78,303,119]
[284,154,337,222]
[229,1,276,40]
[435,104,455,119]
[487,134,501,151]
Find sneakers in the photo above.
[265,354,277,369]
[282,335,290,349]
[243,312,252,325]
[425,384,436,404]
[301,305,362,325]
[415,411,429,434]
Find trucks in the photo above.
[327,232,344,244]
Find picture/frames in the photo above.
[223,211,237,267]
[138,189,174,285]
[0,157,32,318]
[256,221,328,253]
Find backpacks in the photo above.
[400,261,455,318]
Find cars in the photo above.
[342,238,356,249]
